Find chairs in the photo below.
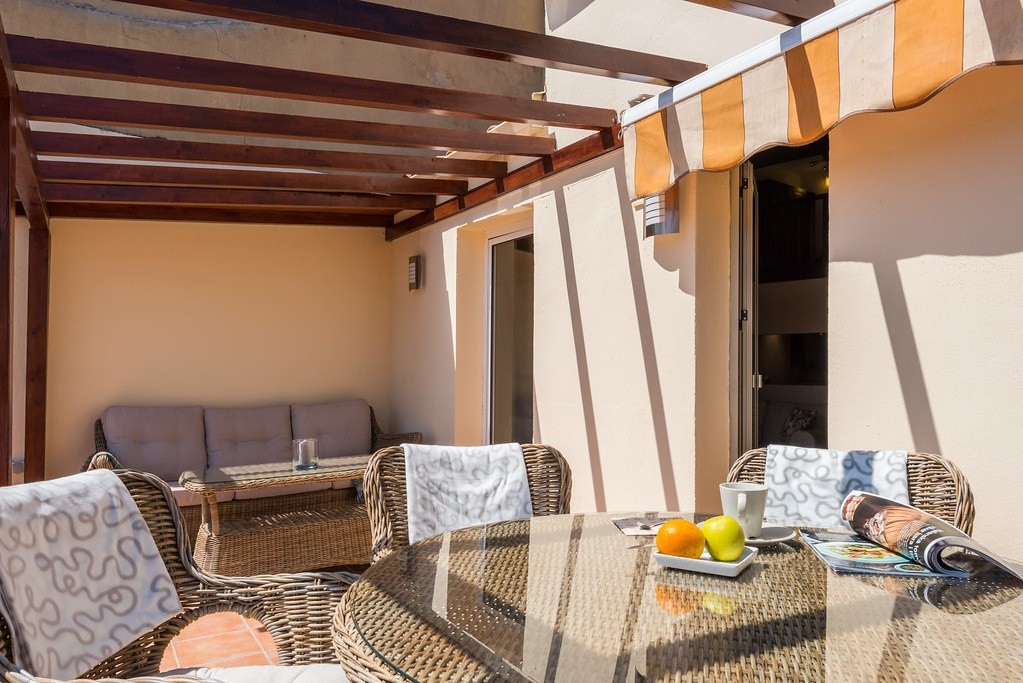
[727,444,976,538]
[362,443,572,565]
[0,469,360,683]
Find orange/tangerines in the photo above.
[656,518,705,559]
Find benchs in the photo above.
[78,405,423,554]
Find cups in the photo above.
[719,482,769,539]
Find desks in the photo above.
[178,454,373,577]
[332,512,1023,683]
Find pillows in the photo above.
[779,405,818,445]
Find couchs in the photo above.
[758,399,828,448]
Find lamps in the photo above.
[643,181,679,241]
[408,255,421,293]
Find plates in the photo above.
[653,545,759,577]
[695,520,796,547]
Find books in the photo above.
[613,518,684,535]
[800,490,1023,582]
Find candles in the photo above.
[291,438,321,472]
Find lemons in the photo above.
[699,516,745,561]
